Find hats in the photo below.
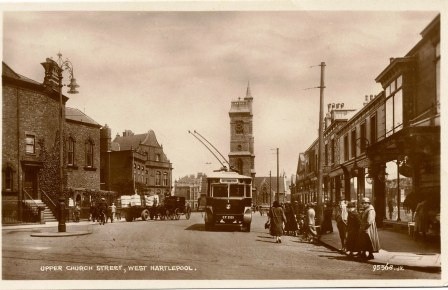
[361,198,370,203]
[347,202,355,207]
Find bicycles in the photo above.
[72,211,79,222]
[100,210,105,225]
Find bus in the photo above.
[204,170,251,231]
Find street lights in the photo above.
[57,61,78,232]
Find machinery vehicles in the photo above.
[116,194,159,221]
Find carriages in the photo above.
[151,196,191,220]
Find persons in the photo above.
[164,189,171,197]
[294,200,299,211]
[253,205,256,212]
[268,201,288,244]
[260,206,264,216]
[88,201,96,223]
[299,202,317,242]
[66,195,75,221]
[109,202,116,222]
[96,198,109,226]
[322,202,334,233]
[282,202,298,238]
[346,203,365,258]
[116,199,123,220]
[411,199,429,242]
[360,198,380,260]
